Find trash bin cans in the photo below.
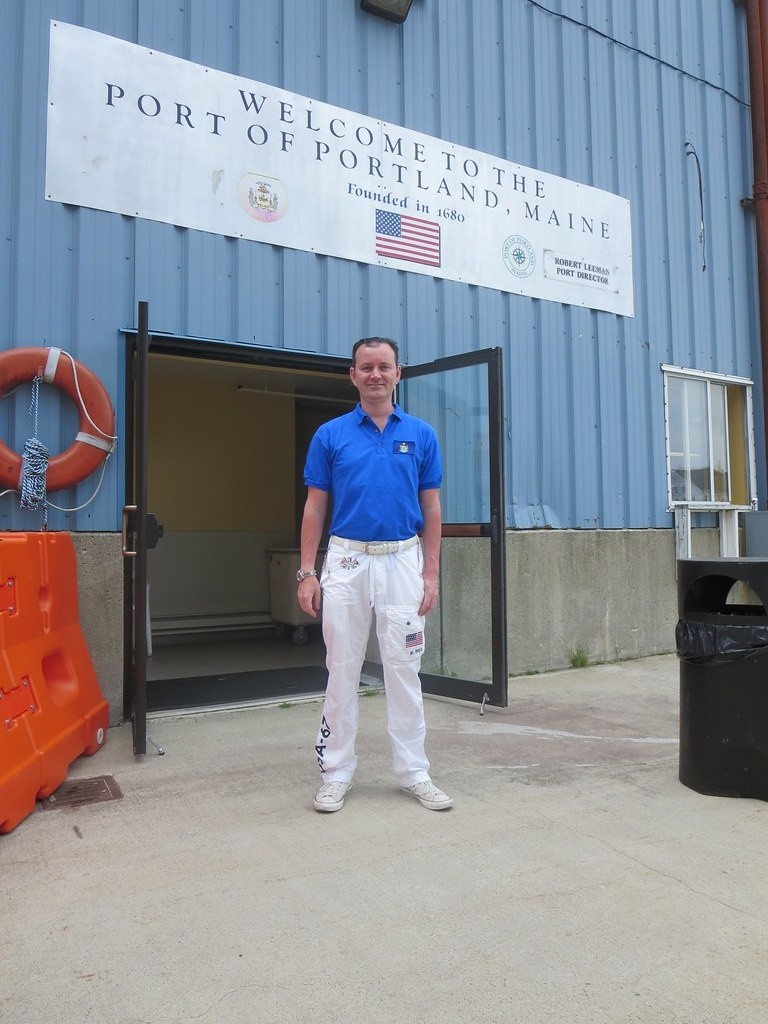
[675,557,768,798]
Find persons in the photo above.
[295,337,454,814]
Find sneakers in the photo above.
[314,782,353,812]
[401,780,454,810]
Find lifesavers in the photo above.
[0,346,116,494]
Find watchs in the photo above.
[296,570,317,581]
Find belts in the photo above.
[331,535,417,556]
